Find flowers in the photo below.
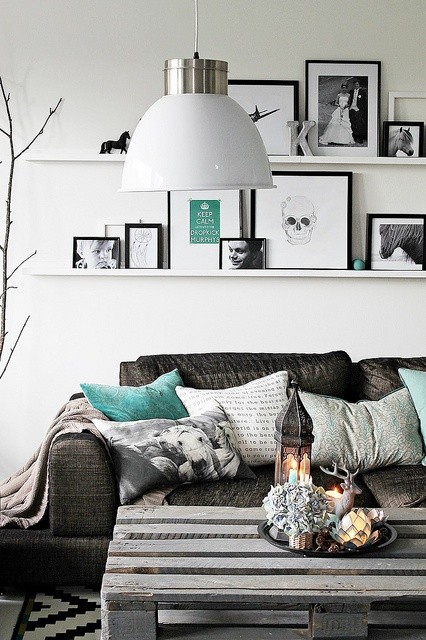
[261,481,337,538]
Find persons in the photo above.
[348,81,368,144]
[75,239,118,269]
[226,241,263,270]
[318,83,355,148]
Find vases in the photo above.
[288,528,313,550]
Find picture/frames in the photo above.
[249,169,353,271]
[218,237,266,270]
[227,78,300,158]
[72,236,121,270]
[304,59,382,160]
[379,119,426,158]
[124,223,162,270]
[365,212,426,274]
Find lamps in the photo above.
[115,0,278,195]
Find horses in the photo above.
[389,125,415,157]
[98,131,130,154]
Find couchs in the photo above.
[1,349,426,588]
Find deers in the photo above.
[320,460,362,519]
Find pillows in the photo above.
[398,367,426,466]
[174,370,290,467]
[297,389,426,474]
[90,396,258,507]
[79,367,190,423]
